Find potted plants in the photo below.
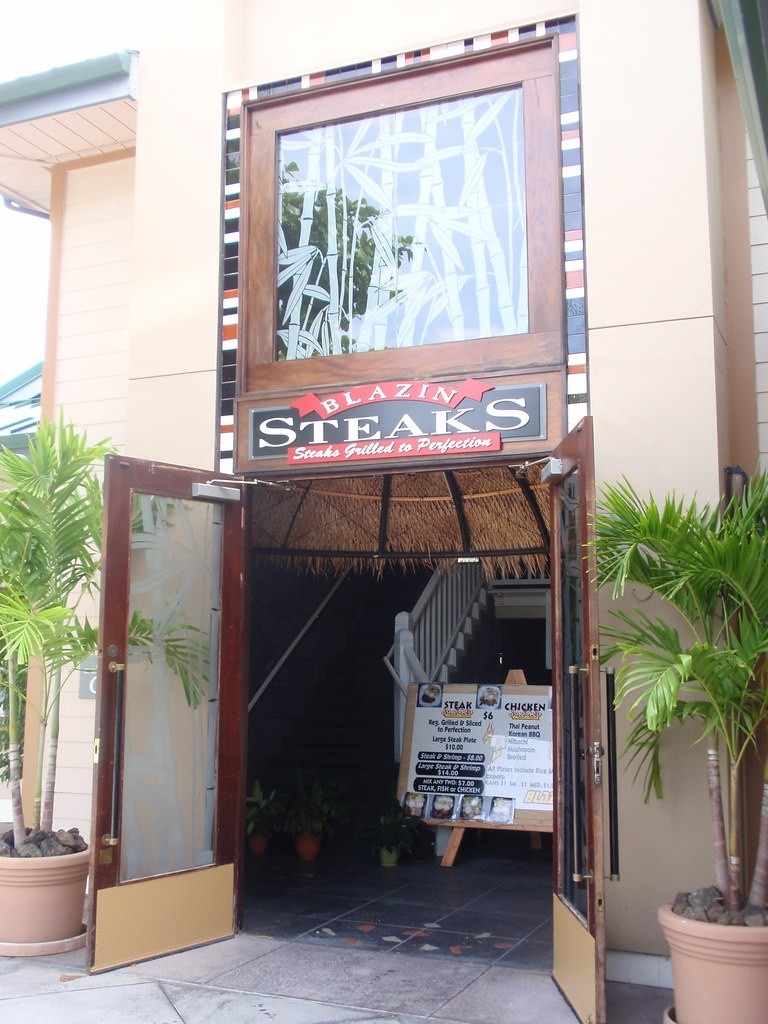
[245,780,286,851]
[0,408,210,963]
[288,768,347,862]
[579,470,768,1024]
[375,797,424,868]
